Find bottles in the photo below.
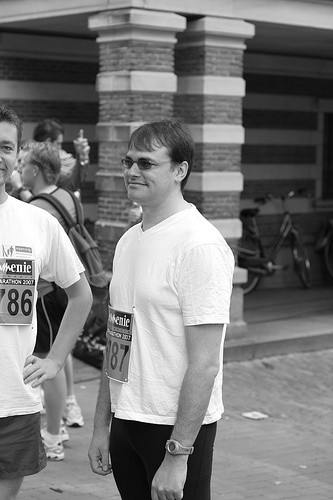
[129,200,142,225]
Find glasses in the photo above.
[120,159,171,171]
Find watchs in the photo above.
[17,186,28,193]
[165,440,194,455]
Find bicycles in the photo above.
[224,186,315,296]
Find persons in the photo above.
[0,105,94,500]
[87,119,235,500]
[17,141,85,460]
[7,118,90,427]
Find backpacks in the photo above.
[26,187,111,338]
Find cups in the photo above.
[73,138,89,166]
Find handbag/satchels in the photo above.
[71,315,107,372]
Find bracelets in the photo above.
[80,160,89,166]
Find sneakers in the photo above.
[39,431,65,461]
[64,399,86,426]
[59,427,69,443]
